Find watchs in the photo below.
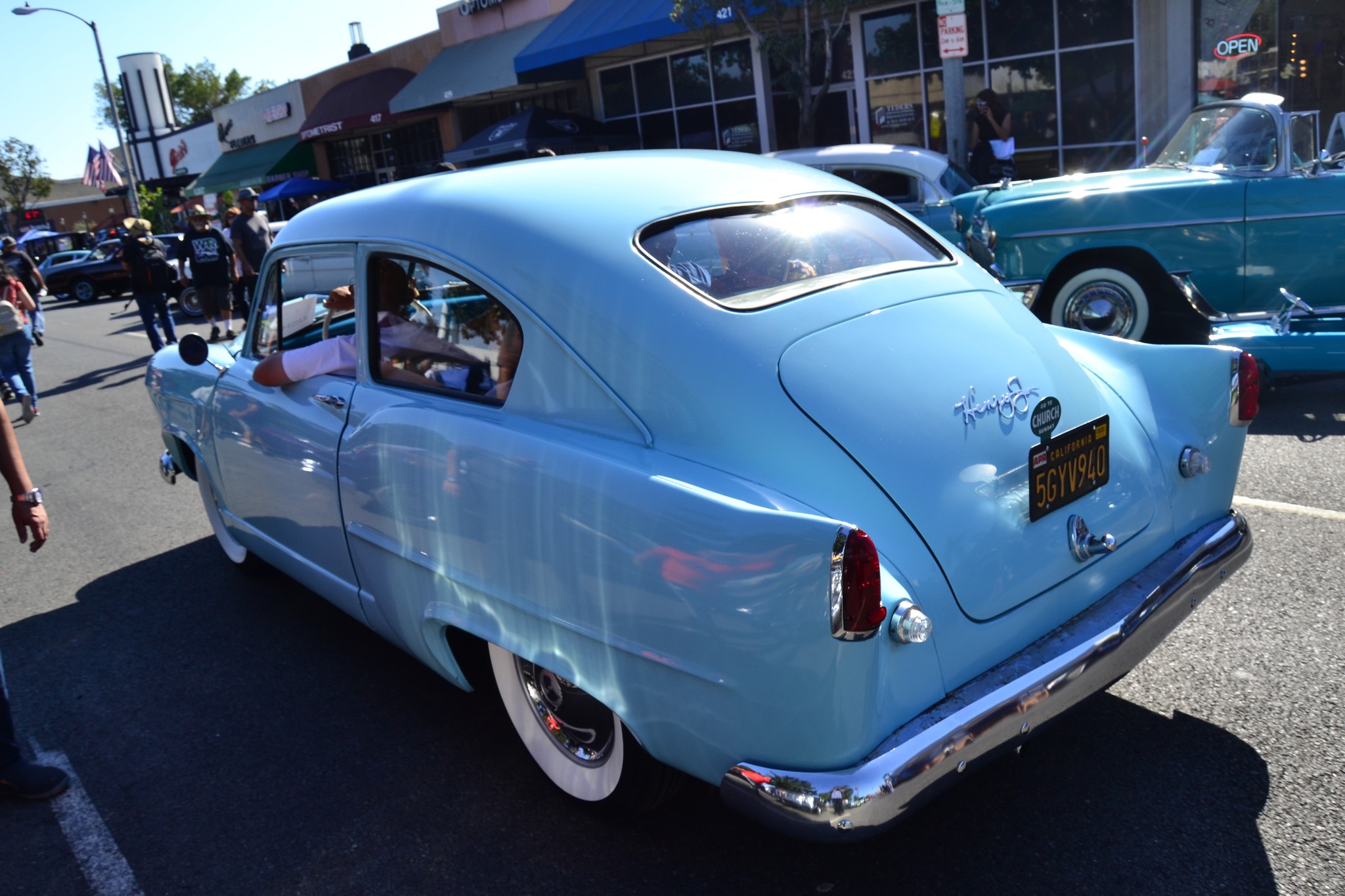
[10,488,43,505]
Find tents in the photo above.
[442,106,625,170]
[255,176,349,223]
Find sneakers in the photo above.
[2,383,15,401]
[226,329,239,340]
[0,757,70,800]
[20,393,35,423]
[210,326,220,342]
[32,330,44,346]
[32,407,41,416]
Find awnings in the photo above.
[388,12,587,116]
[513,0,802,74]
[179,133,320,199]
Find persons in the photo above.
[85,187,322,353]
[0,397,70,800]
[0,235,49,424]
[968,87,1018,185]
[710,220,819,301]
[251,223,712,408]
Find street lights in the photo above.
[11,1,142,221]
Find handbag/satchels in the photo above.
[988,132,1015,160]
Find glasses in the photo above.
[228,214,235,220]
[975,100,986,108]
[247,195,259,203]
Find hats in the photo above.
[349,258,414,306]
[237,186,261,202]
[185,204,213,223]
[123,216,153,245]
[2,236,21,248]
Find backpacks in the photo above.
[0,285,25,338]
[109,236,177,315]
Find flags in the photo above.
[96,141,124,187]
[81,146,107,193]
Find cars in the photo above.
[924,98,1344,344]
[145,148,1260,835]
[34,218,290,319]
[758,138,973,213]
[1205,285,1344,395]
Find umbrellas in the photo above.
[296,68,453,183]
